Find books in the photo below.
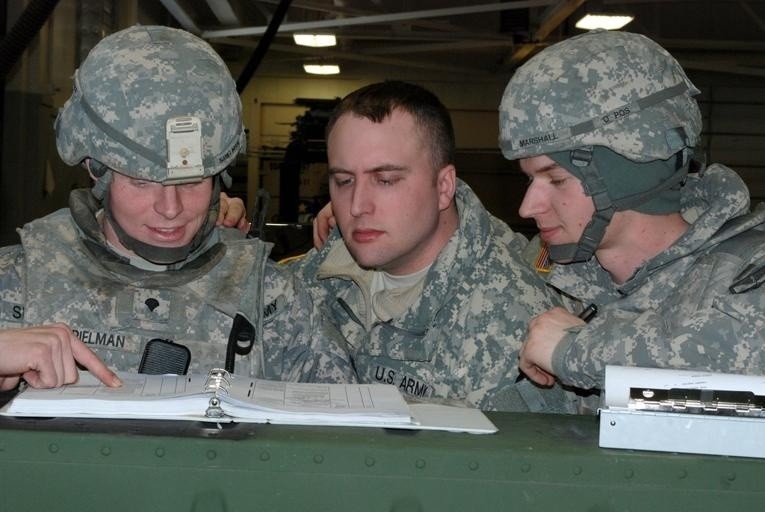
[0,366,417,438]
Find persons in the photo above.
[214,78,582,416]
[0,24,359,425]
[310,25,764,414]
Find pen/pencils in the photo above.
[515,303,598,383]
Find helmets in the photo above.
[499,27,705,163]
[54,25,247,186]
